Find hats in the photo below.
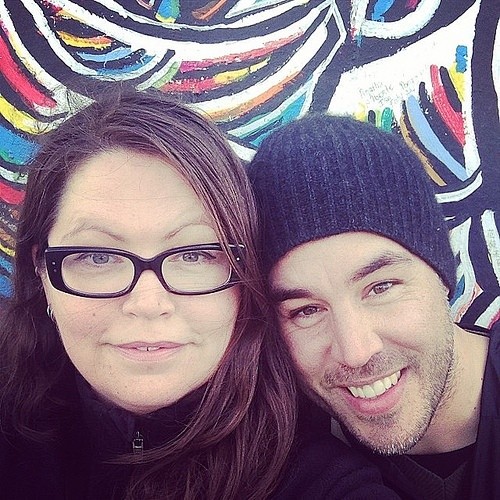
[247,114,459,301]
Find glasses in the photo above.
[43,241,247,299]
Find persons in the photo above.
[248,104,499,500]
[2,87,406,500]
